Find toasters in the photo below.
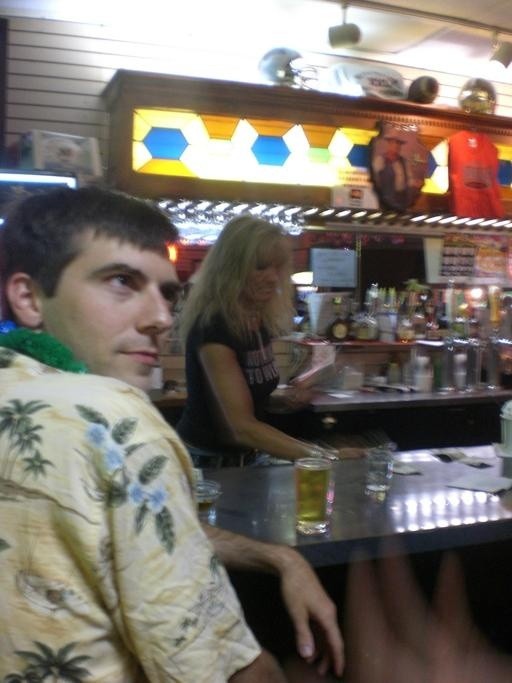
[413,357,435,400]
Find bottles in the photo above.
[402,347,423,387]
[191,455,205,488]
[451,353,474,395]
[386,362,402,392]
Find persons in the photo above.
[373,126,419,208]
[1,185,344,683]
[172,215,376,470]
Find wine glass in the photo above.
[465,307,492,391]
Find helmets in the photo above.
[259,47,303,90]
[458,78,497,115]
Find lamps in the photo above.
[318,0,512,73]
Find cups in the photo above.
[295,458,333,536]
[362,448,395,492]
[148,366,164,390]
[492,401,511,459]
[192,481,222,527]
